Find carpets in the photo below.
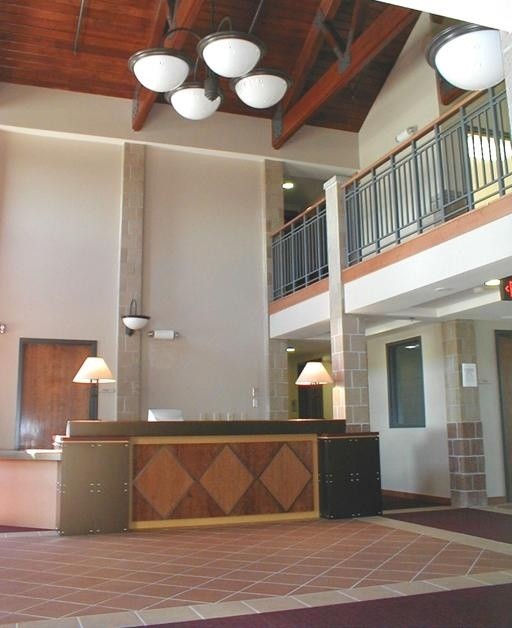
[131,583,512,626]
[382,508,512,545]
[0,525,46,534]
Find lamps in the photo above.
[424,12,503,92]
[296,361,333,422]
[125,0,293,123]
[72,355,115,422]
[120,301,150,337]
